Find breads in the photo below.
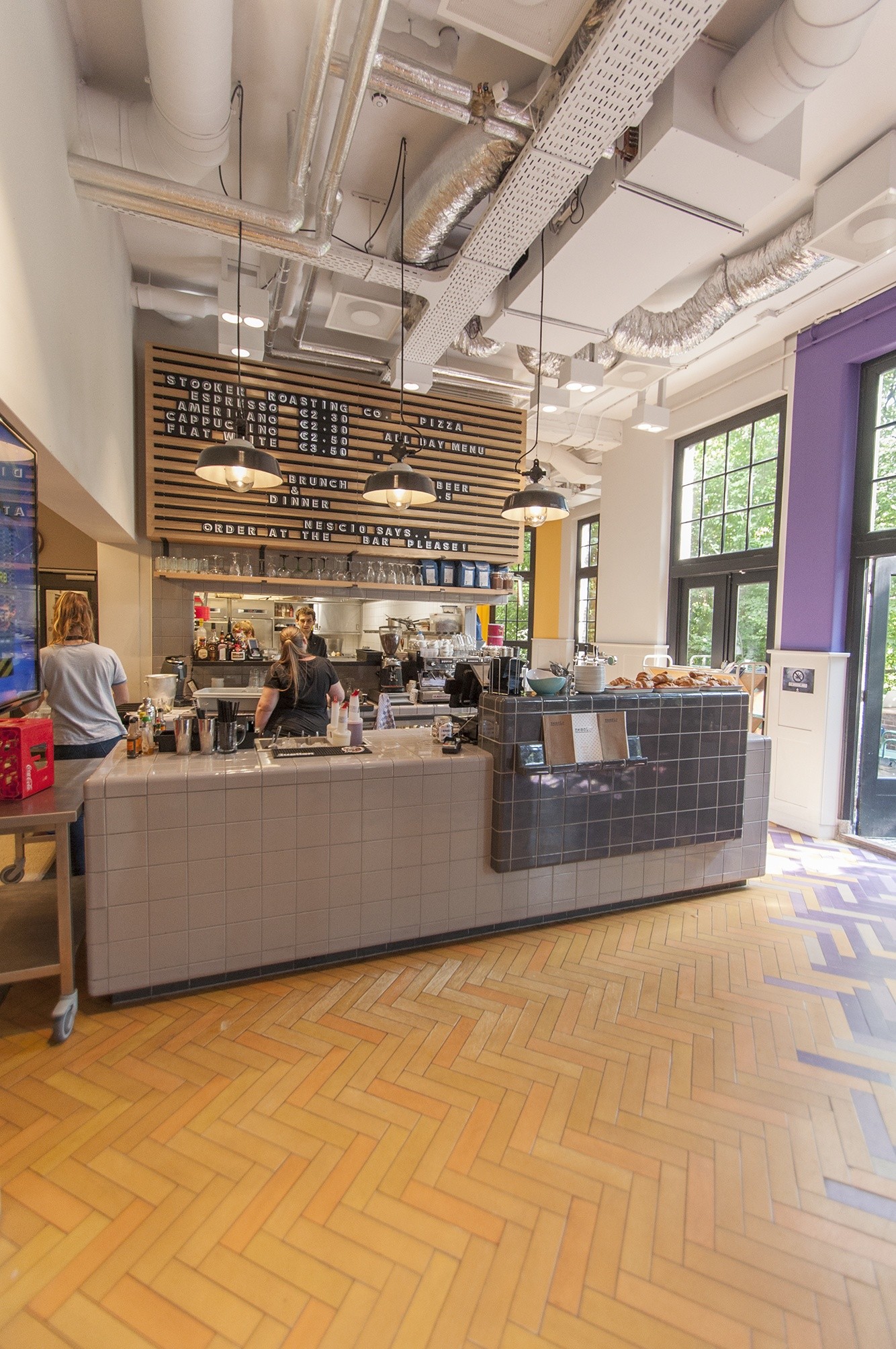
[610,671,734,688]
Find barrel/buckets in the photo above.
[144,674,179,711]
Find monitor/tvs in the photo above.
[0,415,44,716]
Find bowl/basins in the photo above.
[525,669,566,694]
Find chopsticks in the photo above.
[216,699,240,722]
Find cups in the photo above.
[197,716,216,754]
[173,718,192,755]
[217,721,246,753]
[408,640,454,657]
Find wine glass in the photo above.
[209,550,425,586]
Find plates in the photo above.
[573,665,605,694]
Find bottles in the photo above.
[417,630,424,642]
[490,572,514,590]
[274,603,294,617]
[126,698,166,759]
[195,618,236,661]
[326,689,363,747]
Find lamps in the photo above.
[192,81,285,493]
[361,137,438,512]
[501,227,571,527]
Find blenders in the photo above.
[379,627,405,693]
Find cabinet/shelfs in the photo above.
[151,570,513,666]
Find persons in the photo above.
[21,590,128,877]
[232,621,254,644]
[296,607,327,657]
[254,626,345,739]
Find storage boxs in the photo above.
[0,717,54,802]
